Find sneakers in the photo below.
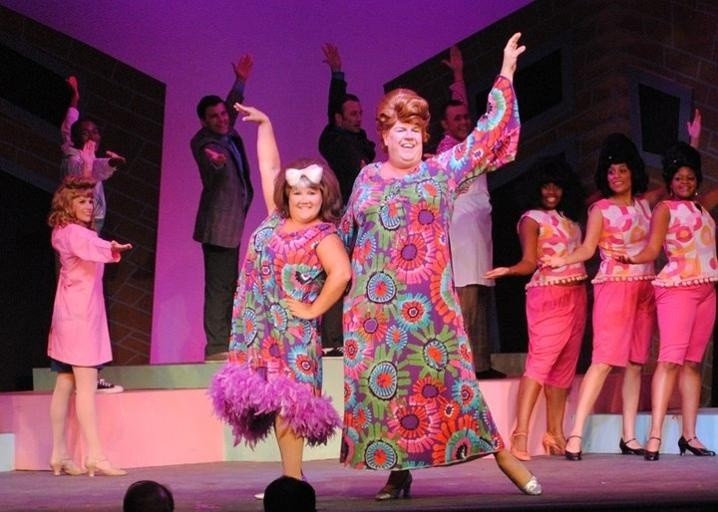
[205,352,227,363]
[97,379,123,393]
[322,347,343,359]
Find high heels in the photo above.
[620,437,661,460]
[375,471,412,500]
[50,457,87,475]
[522,474,542,495]
[678,435,715,456]
[86,456,127,476]
[511,432,530,461]
[543,433,582,460]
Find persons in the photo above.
[434,42,508,380]
[611,140,718,459]
[338,31,544,501]
[47,175,134,479]
[123,478,174,510]
[189,52,256,365]
[57,74,123,394]
[539,107,704,461]
[318,39,376,356]
[233,102,354,500]
[264,475,316,512]
[480,172,589,462]
